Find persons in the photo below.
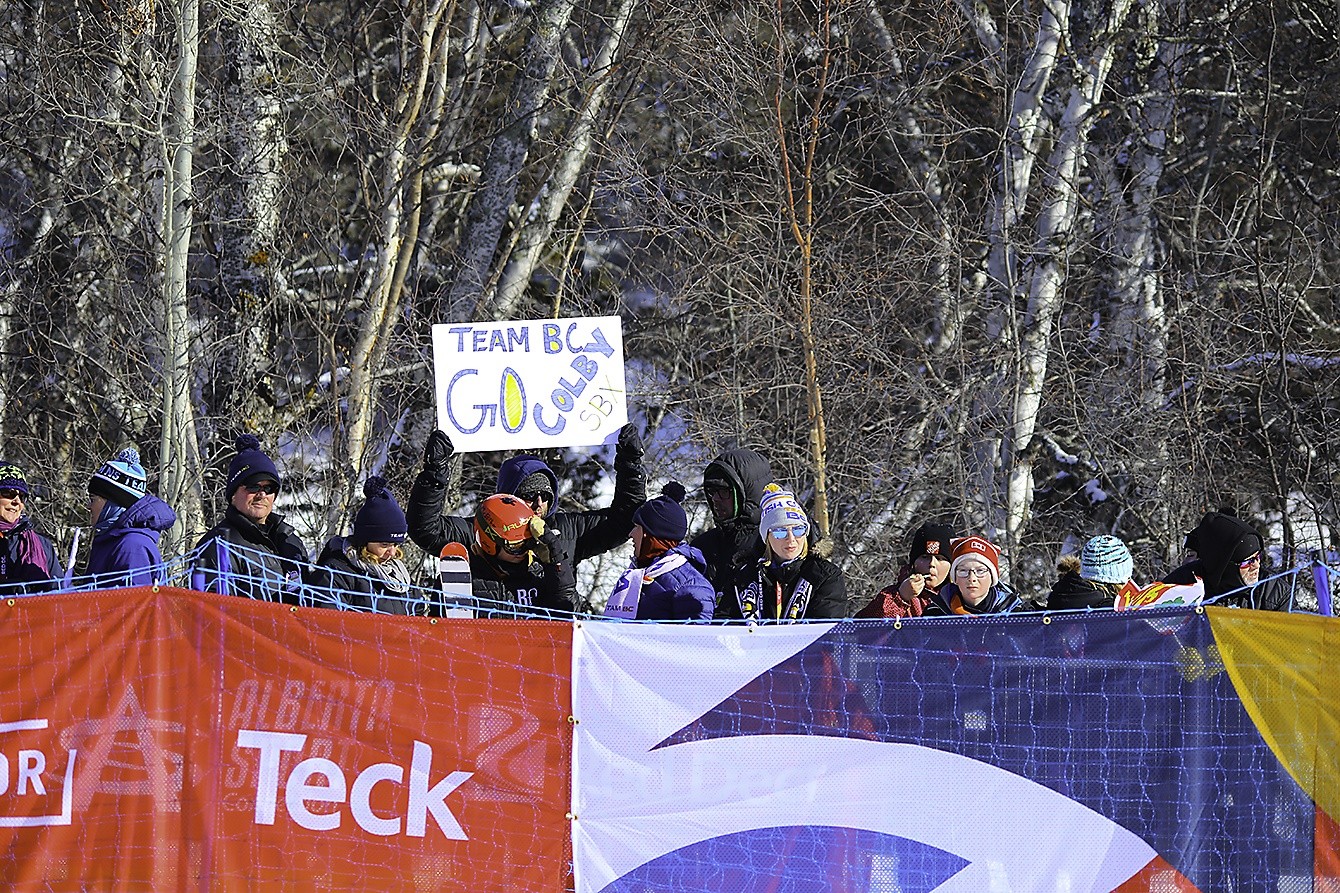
[82,449,176,588]
[0,462,65,595]
[687,449,774,617]
[1045,535,1132,610]
[601,481,716,625]
[1162,511,1293,613]
[428,493,581,620]
[920,536,1023,617]
[307,476,424,616]
[405,424,646,586]
[190,435,310,606]
[708,484,847,624]
[854,525,954,617]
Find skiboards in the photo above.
[436,541,474,620]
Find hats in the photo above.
[89,447,146,508]
[0,460,31,495]
[516,470,555,513]
[908,523,957,567]
[949,536,1001,587]
[632,482,688,541]
[704,466,734,489]
[225,434,281,502]
[1231,534,1262,562]
[759,483,810,544]
[353,476,407,546]
[1083,535,1133,583]
[1182,527,1199,552]
[244,473,280,487]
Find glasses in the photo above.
[1239,551,1262,569]
[246,484,279,494]
[0,487,30,504]
[518,490,551,502]
[501,538,538,555]
[704,485,732,500]
[769,525,808,539]
[955,566,990,578]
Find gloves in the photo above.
[423,430,454,472]
[617,423,645,465]
[530,514,577,596]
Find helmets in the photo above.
[473,494,534,556]
